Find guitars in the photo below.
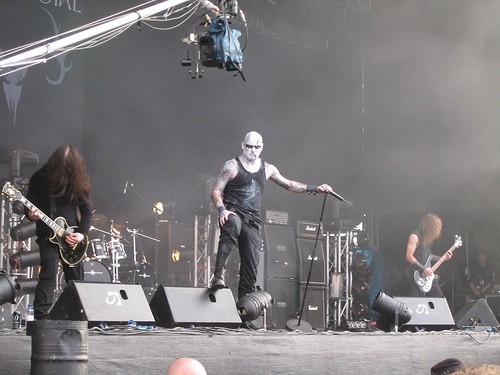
[412,234,464,293]
[2,181,89,267]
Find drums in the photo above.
[56,238,127,293]
[119,263,158,297]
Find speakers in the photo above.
[377,295,500,331]
[261,224,326,331]
[47,279,242,329]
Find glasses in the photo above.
[243,144,263,149]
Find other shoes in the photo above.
[240,320,256,330]
[213,278,225,287]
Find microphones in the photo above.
[330,192,344,201]
[124,181,128,194]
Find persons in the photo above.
[405,213,454,297]
[167,358,207,375]
[25,144,94,321]
[467,250,497,302]
[210,130,332,329]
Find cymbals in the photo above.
[91,214,108,229]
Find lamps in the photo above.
[371,292,412,332]
[236,285,275,330]
[340,320,368,332]
[152,199,178,222]
[172,248,200,287]
[0,200,40,305]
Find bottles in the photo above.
[25,304,34,329]
[13,310,21,329]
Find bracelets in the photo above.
[307,184,317,194]
[217,206,225,213]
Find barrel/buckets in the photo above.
[30,320,89,375]
